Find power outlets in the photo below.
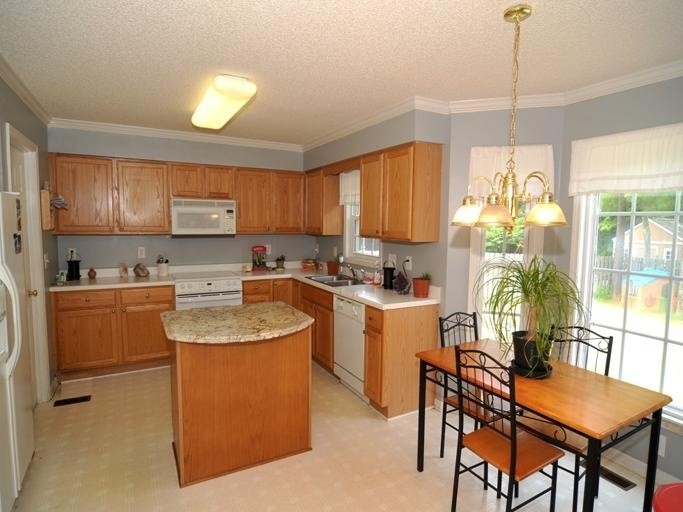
[404,256,411,270]
[67,248,75,260]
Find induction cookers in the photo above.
[173,270,240,282]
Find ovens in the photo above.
[176,291,244,309]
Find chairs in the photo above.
[449,343,564,512]
[513,321,617,512]
[437,307,525,489]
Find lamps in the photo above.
[187,74,259,133]
[449,4,567,234]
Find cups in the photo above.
[383,268,396,290]
[157,264,168,276]
[67,260,80,280]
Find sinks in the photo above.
[325,280,366,287]
[308,275,353,281]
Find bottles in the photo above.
[373,262,380,284]
[88,269,96,278]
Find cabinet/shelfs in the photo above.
[299,281,332,375]
[234,165,302,236]
[48,283,176,382]
[302,165,343,236]
[273,279,293,306]
[365,303,440,420]
[46,151,168,237]
[242,279,270,304]
[360,138,443,244]
[167,160,235,199]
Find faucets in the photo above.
[346,262,358,280]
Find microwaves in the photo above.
[172,200,235,235]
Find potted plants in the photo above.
[470,251,593,380]
[326,254,339,275]
[274,254,285,268]
[409,270,432,298]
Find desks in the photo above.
[413,339,670,512]
[159,301,315,490]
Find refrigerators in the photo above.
[1,191,36,512]
